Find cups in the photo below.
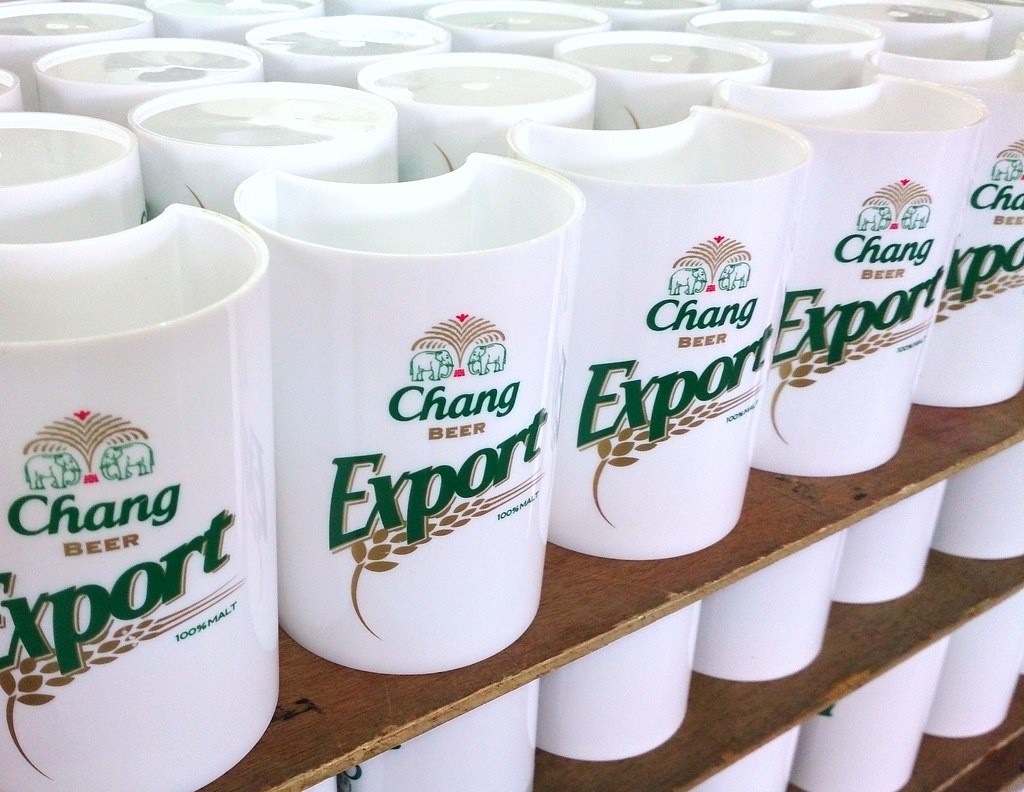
[1,1,1024,791]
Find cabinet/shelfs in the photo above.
[191,387,1024,792]
